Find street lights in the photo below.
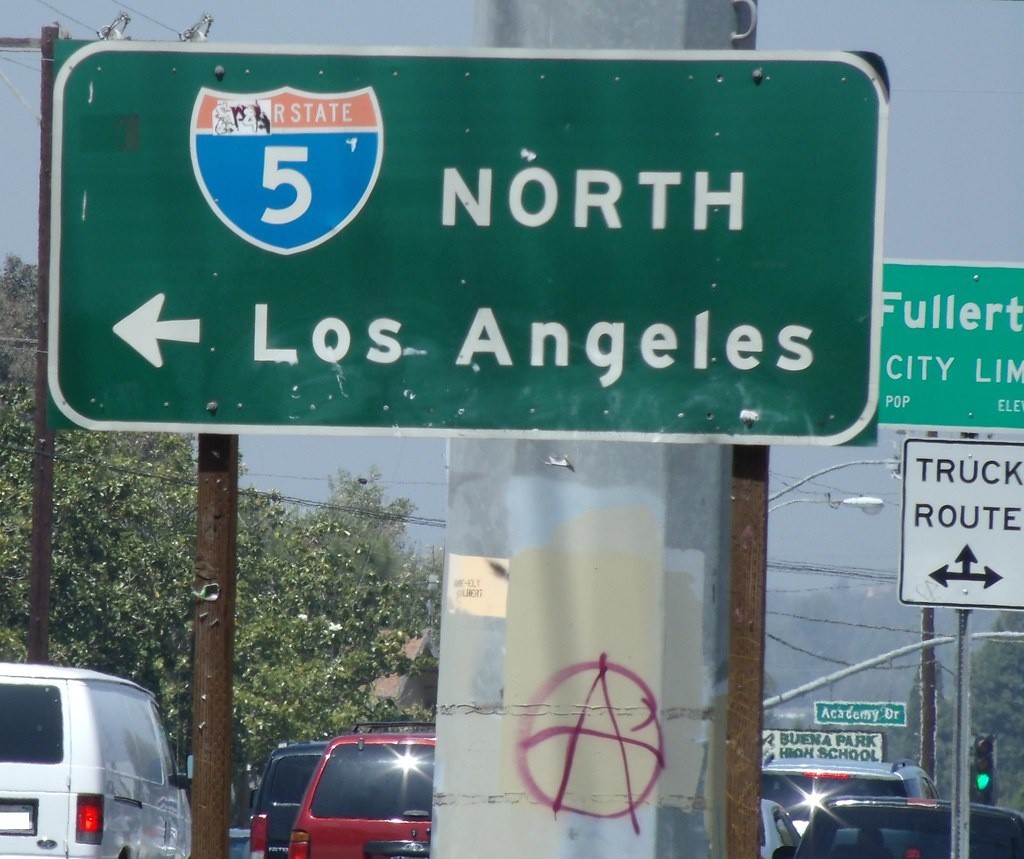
[769,493,882,535]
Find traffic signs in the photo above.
[48,43,886,447]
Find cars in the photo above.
[0,656,194,856]
[760,753,937,858]
[770,792,1024,859]
[291,723,434,859]
[250,737,334,859]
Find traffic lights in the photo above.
[970,734,997,803]
